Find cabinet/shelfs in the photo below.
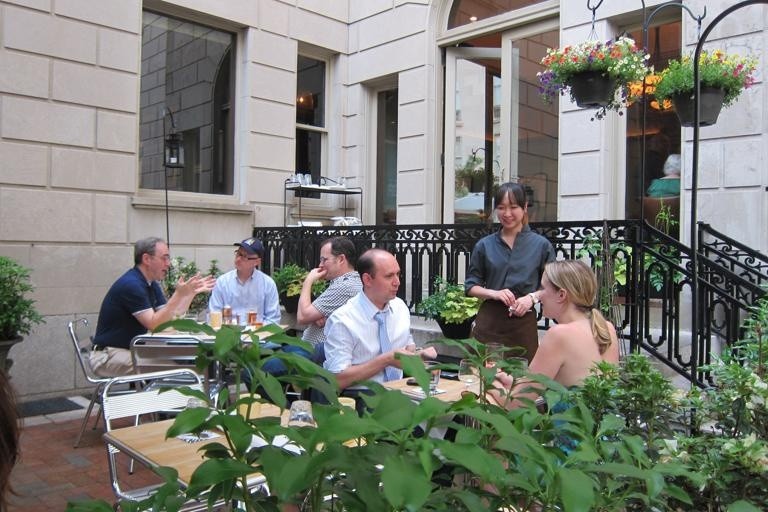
[284,176,362,227]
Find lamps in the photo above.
[162,106,185,248]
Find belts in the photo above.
[92,345,106,350]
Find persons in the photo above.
[244,237,362,411]
[321,248,437,391]
[465,182,553,366]
[205,239,282,359]
[646,153,680,197]
[481,260,619,511]
[88,236,216,380]
[0,366,28,510]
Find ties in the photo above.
[374,314,399,381]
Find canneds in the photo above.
[222,305,232,325]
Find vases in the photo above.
[672,88,725,127]
[571,72,618,108]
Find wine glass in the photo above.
[458,358,480,392]
[335,175,347,188]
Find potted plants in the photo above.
[0,256,46,380]
[414,275,482,341]
[455,155,499,198]
[272,262,328,314]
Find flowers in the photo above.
[651,49,758,107]
[536,36,649,121]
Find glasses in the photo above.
[234,251,257,260]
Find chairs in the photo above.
[101,369,233,512]
[68,317,209,512]
[637,195,679,227]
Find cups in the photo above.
[175,306,261,333]
[187,392,357,435]
[507,356,530,382]
[484,342,505,374]
[423,361,442,395]
[290,173,311,186]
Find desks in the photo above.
[149,325,290,344]
[378,371,545,417]
[101,402,370,501]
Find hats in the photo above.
[234,238,263,253]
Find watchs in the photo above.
[527,293,539,304]
